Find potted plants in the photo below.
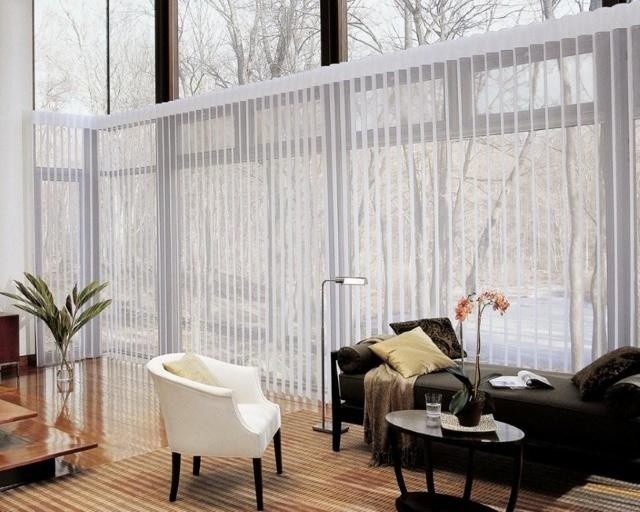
[0,270,112,392]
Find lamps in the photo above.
[309,275,369,435]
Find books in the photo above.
[488,369,554,389]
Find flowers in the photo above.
[447,283,511,416]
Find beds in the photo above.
[331,333,640,484]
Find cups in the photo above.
[425,415,441,436]
[425,393,443,418]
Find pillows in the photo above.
[566,344,640,401]
[162,350,225,388]
[336,342,385,375]
[387,316,469,360]
[366,325,458,380]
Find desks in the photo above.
[1,311,20,378]
[0,397,99,493]
[383,409,528,511]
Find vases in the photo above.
[454,399,486,427]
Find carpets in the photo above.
[0,408,639,512]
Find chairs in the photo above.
[145,351,283,512]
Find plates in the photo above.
[442,413,498,435]
